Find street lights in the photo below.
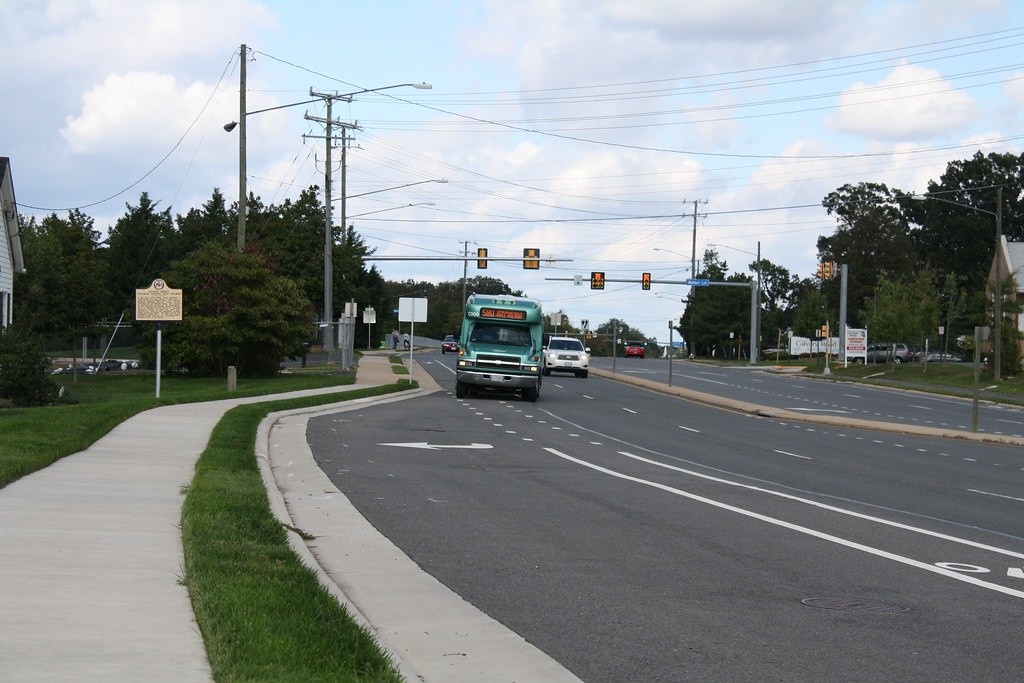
[910,189,1003,383]
[321,179,451,353]
[704,239,761,364]
[339,201,438,246]
[653,246,696,358]
[221,80,436,253]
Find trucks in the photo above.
[453,290,549,402]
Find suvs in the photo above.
[441,334,461,354]
[624,343,646,359]
[543,335,591,378]
[848,342,912,364]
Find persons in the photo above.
[403,332,411,348]
[391,328,399,349]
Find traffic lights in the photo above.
[590,271,605,290]
[642,272,651,290]
[824,262,833,280]
[832,262,840,278]
[816,262,823,279]
[821,325,827,337]
